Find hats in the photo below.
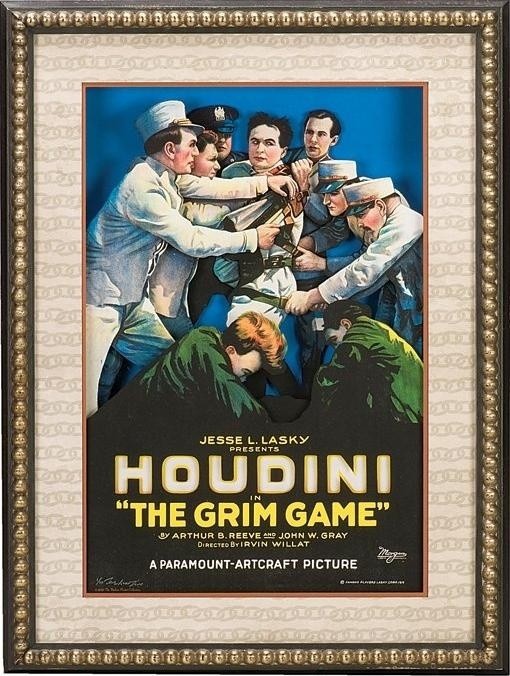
[131,99,206,143]
[313,160,357,194]
[336,177,395,219]
[184,105,239,133]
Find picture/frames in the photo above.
[0,0,510,676]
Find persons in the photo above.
[85,100,424,428]
